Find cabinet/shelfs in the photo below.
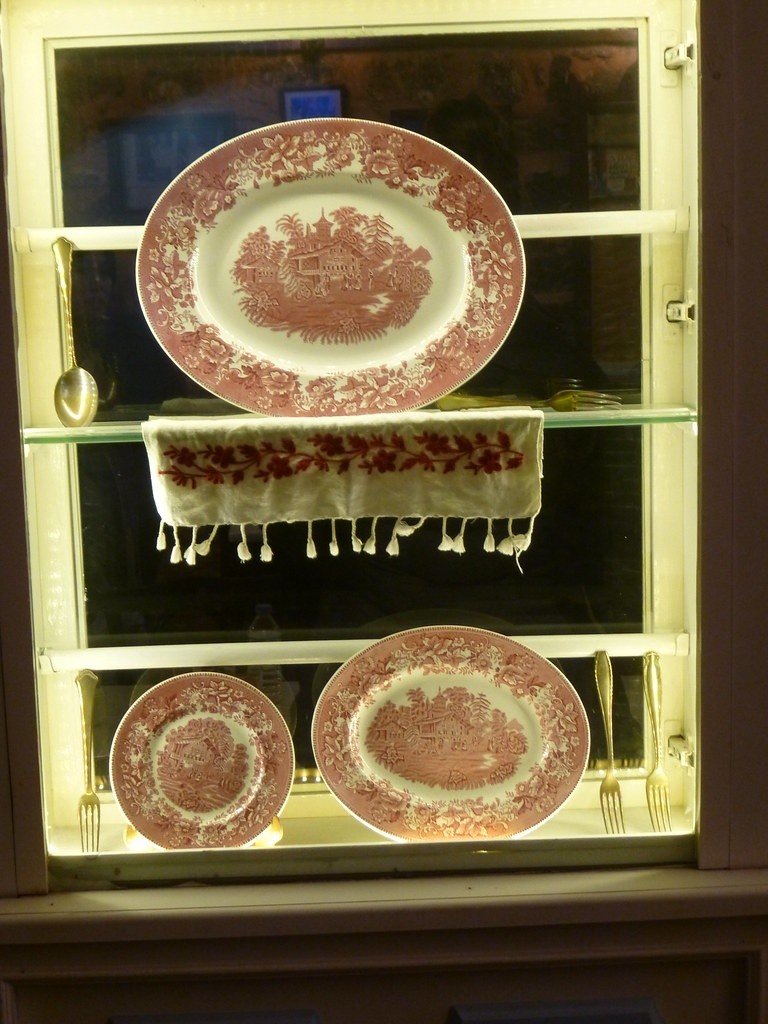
[0,0,700,885]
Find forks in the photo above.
[435,389,623,412]
[72,667,102,856]
[594,650,625,834]
[642,651,672,832]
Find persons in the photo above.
[423,97,504,176]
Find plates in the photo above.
[134,117,527,418]
[108,671,296,850]
[311,625,591,843]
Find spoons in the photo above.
[51,237,99,428]
